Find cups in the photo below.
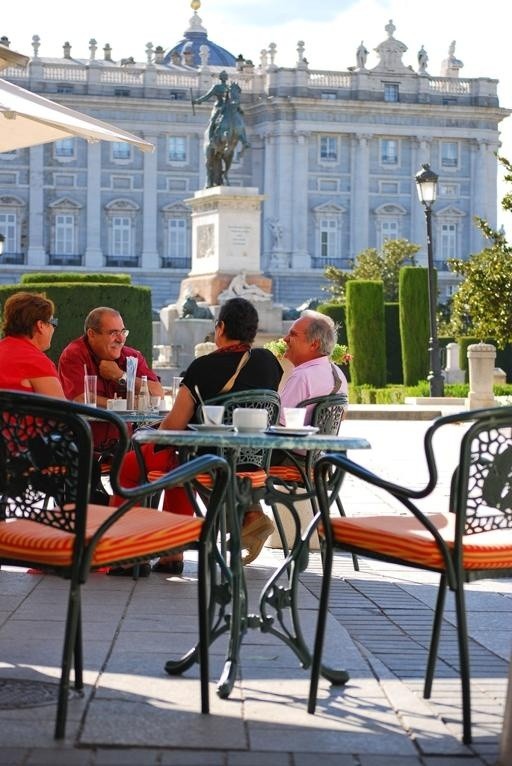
[202,405,225,424]
[164,395,174,411]
[284,407,306,429]
[84,375,98,411]
[106,398,127,411]
[172,377,184,411]
[151,397,161,412]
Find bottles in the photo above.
[138,375,150,413]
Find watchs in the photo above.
[117,372,129,385]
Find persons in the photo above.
[416,44,429,75]
[1,292,114,507]
[224,309,350,565]
[356,39,370,69]
[59,306,167,460]
[385,18,396,38]
[192,69,252,153]
[107,298,283,579]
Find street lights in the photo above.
[413,162,451,394]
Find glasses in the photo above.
[92,330,129,337]
[40,318,58,327]
[214,319,221,327]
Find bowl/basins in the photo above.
[233,407,268,431]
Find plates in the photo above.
[269,425,319,435]
[104,410,136,415]
[188,424,234,433]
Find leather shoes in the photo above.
[107,563,151,577]
[152,561,183,574]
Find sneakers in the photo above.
[242,526,274,564]
[227,516,273,552]
